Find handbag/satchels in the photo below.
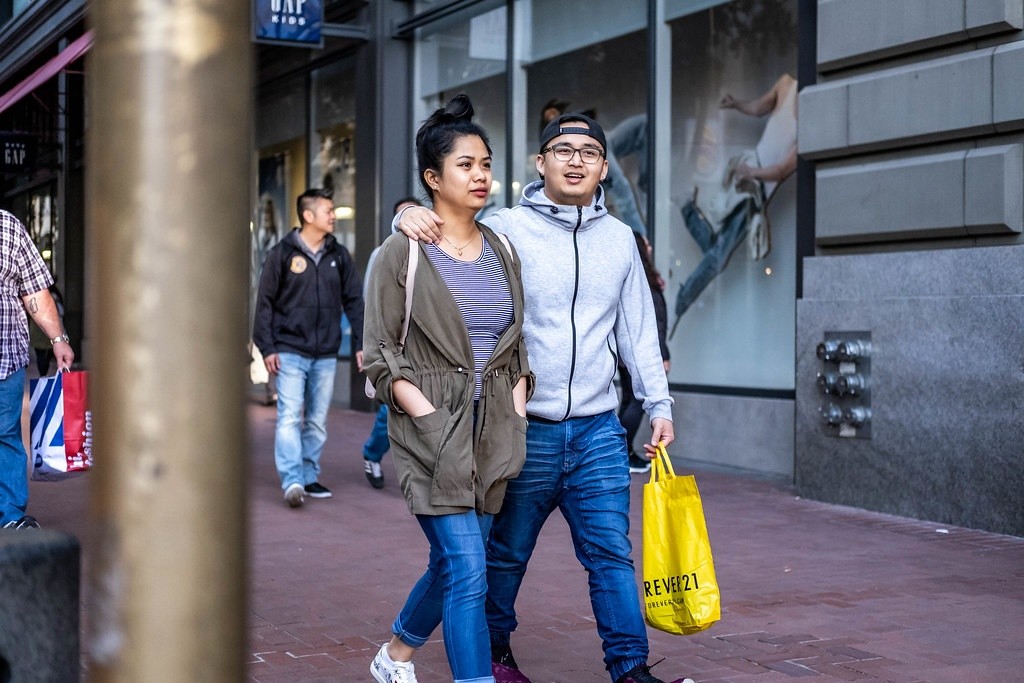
[643,442,721,635]
[28,366,93,481]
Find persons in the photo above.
[363,197,422,488]
[251,189,364,506]
[539,99,648,236]
[28,273,64,376]
[617,232,671,473]
[672,76,801,346]
[360,117,538,683]
[392,111,694,683]
[257,198,278,257]
[0,208,74,530]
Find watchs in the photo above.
[50,333,69,345]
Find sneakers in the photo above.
[370,643,417,683]
[614,657,696,683]
[364,457,384,489]
[628,452,651,473]
[492,661,532,683]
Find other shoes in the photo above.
[285,483,304,507]
[304,483,332,498]
[4,515,41,529]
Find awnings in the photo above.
[0,28,97,112]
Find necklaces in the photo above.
[439,230,477,255]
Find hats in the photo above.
[539,113,607,159]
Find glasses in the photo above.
[540,144,604,164]
